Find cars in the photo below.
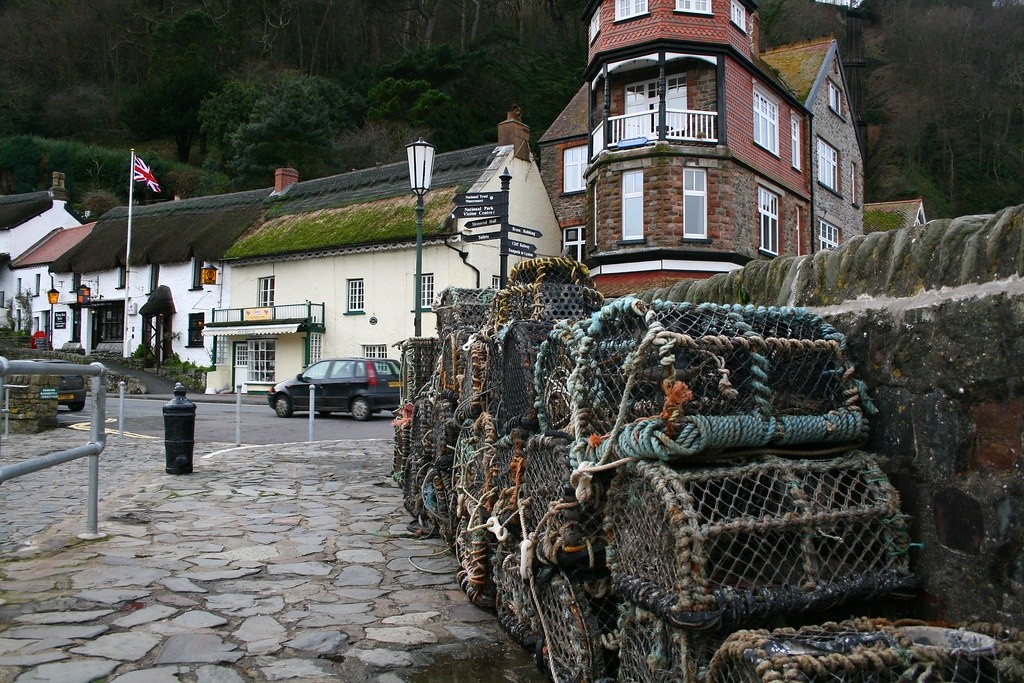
[267,357,404,421]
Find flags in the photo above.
[134,156,161,192]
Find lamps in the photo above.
[200,264,218,285]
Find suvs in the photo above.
[30,359,86,411]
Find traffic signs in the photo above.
[452,191,544,259]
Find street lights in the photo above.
[404,137,437,390]
